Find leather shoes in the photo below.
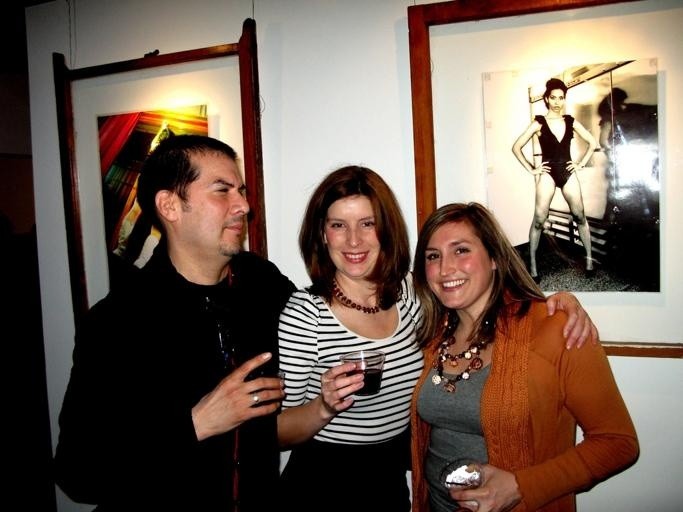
[585,263,595,278]
[529,267,541,285]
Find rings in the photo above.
[252,393,260,404]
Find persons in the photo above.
[409,198,640,512]
[511,77,598,285]
[594,87,651,246]
[277,164,601,512]
[47,133,299,512]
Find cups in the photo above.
[437,456,486,490]
[252,367,285,415]
[341,350,387,396]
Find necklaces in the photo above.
[428,314,494,393]
[330,280,380,316]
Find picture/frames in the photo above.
[404,2,682,361]
[48,18,270,341]
